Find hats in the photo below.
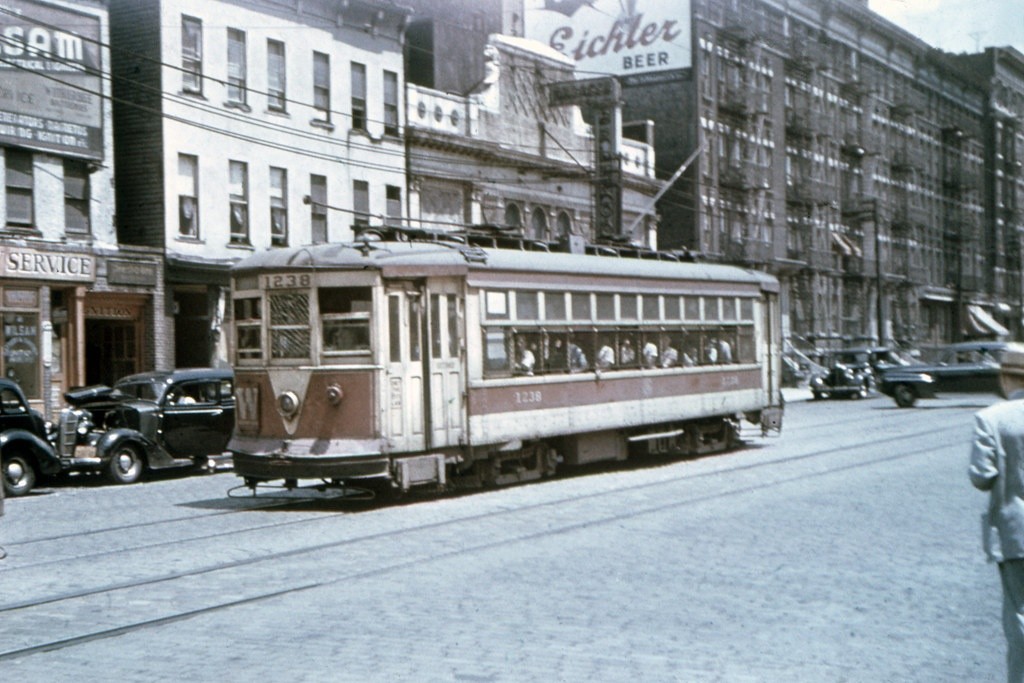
[982,341,1024,373]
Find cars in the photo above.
[875,338,1024,408]
[52,366,241,486]
[810,361,875,399]
[0,378,61,495]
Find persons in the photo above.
[966,342,1024,683]
[513,331,736,376]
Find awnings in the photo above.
[968,304,1011,336]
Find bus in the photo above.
[225,216,789,502]
